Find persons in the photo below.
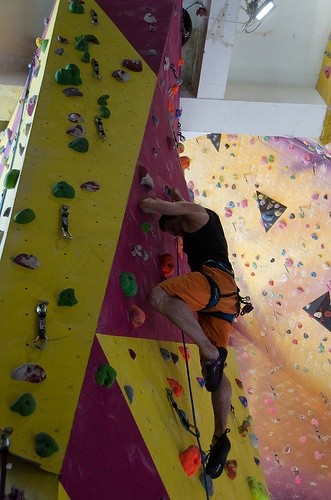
[140,186,242,479]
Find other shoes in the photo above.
[205,428,231,479]
[205,347,227,392]
